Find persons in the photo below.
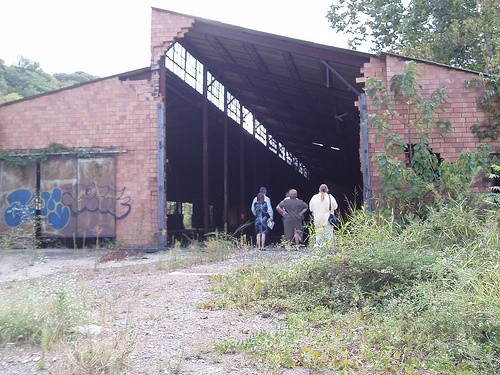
[276,189,309,252]
[308,183,339,255]
[250,186,275,247]
[253,193,269,252]
[276,190,306,247]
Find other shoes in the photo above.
[258,248,267,251]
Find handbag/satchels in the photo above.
[329,214,340,227]
[266,218,275,230]
